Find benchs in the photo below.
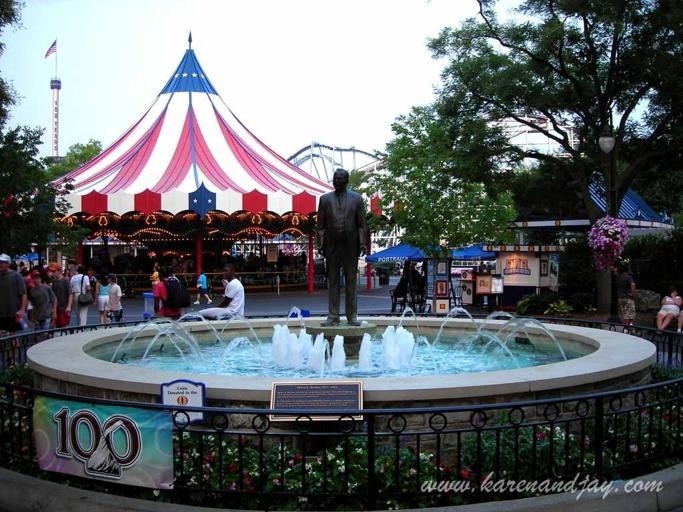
[653,314,683,331]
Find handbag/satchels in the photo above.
[79,293,95,306]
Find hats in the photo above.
[30,269,40,276]
[0,253,12,264]
[48,262,60,271]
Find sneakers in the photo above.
[207,300,212,304]
[194,301,200,304]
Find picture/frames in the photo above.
[436,280,448,297]
[436,261,447,276]
[540,260,548,276]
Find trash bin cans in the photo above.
[376,267,389,285]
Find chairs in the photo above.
[389,289,426,313]
[449,286,464,308]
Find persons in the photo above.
[656,288,683,334]
[150,263,246,319]
[614,267,636,334]
[677,309,683,334]
[397,262,427,312]
[0,254,123,368]
[317,167,370,326]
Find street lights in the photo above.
[598,121,622,324]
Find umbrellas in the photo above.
[365,240,441,261]
[451,243,495,261]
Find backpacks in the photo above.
[165,278,191,307]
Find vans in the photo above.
[452,260,475,267]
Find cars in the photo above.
[490,260,497,267]
